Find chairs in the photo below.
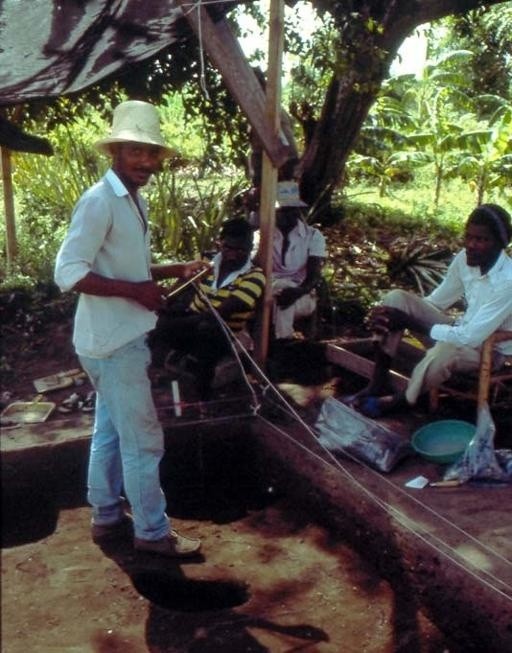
[449,333,512,407]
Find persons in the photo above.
[53,99,213,555]
[158,218,267,360]
[344,204,512,416]
[250,180,327,341]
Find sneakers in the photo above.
[135,532,202,556]
[91,512,133,538]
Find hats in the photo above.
[92,100,180,159]
[274,181,308,209]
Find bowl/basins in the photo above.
[411,419,478,466]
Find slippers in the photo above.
[58,389,96,413]
